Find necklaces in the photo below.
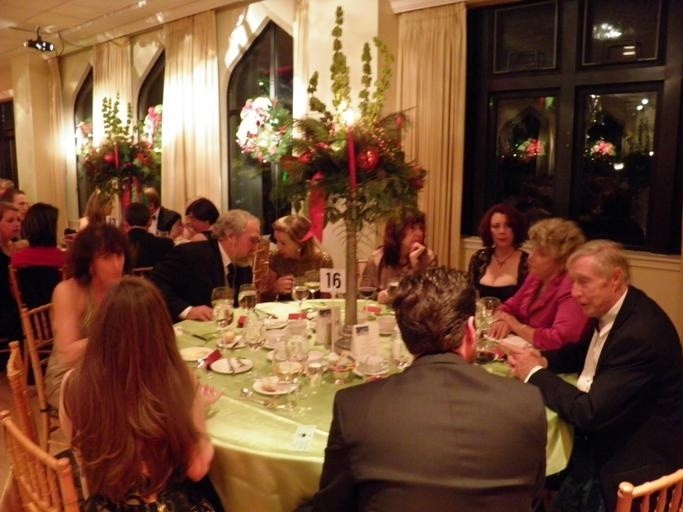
[493,248,516,270]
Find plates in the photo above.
[178,315,412,395]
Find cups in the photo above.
[473,336,502,363]
[157,230,168,237]
[470,296,502,331]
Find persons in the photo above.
[502,239,682,512]
[54,275,225,511]
[464,203,533,309]
[316,263,550,511]
[45,222,132,411]
[359,205,437,305]
[0,174,219,384]
[482,216,594,353]
[151,209,262,323]
[261,215,333,301]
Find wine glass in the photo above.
[210,283,258,347]
[357,277,377,314]
[288,276,309,320]
[303,269,321,310]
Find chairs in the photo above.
[7,340,40,446]
[0,409,80,512]
[20,303,72,452]
[131,266,153,278]
[616,469,682,512]
[9,265,65,311]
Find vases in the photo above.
[334,213,356,360]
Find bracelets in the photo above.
[515,324,527,335]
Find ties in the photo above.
[228,264,236,286]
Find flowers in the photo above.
[239,13,427,235]
[77,89,163,209]
